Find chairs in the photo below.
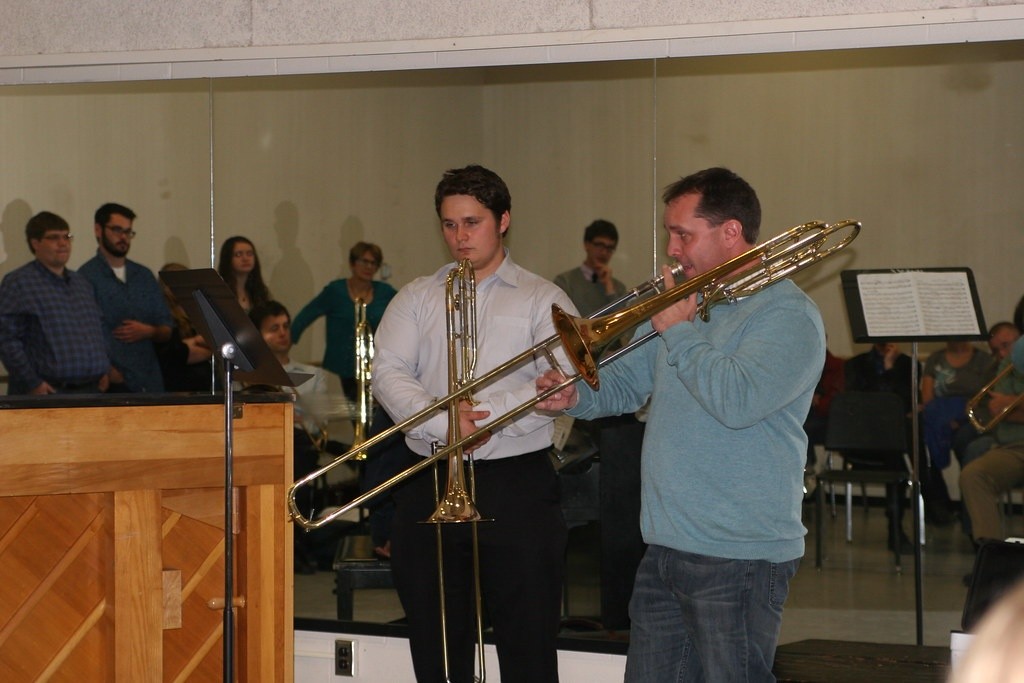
[558,460,600,617]
[815,391,908,572]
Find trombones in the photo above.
[277,380,331,454]
[417,254,488,683]
[284,217,864,535]
[345,297,378,464]
[965,361,1024,434]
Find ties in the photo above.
[592,273,598,282]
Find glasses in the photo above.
[101,225,136,239]
[589,241,616,254]
[35,233,74,243]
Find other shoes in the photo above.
[887,533,914,554]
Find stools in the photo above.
[334,535,396,621]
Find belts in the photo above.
[49,381,98,392]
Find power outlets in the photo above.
[335,640,355,677]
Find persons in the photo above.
[555,219,626,318]
[291,242,397,403]
[959,322,1024,586]
[155,264,217,393]
[218,236,271,313]
[373,165,581,683]
[0,211,112,396]
[843,342,923,554]
[250,300,345,435]
[915,340,993,530]
[76,202,179,395]
[803,348,847,464]
[534,167,826,683]
